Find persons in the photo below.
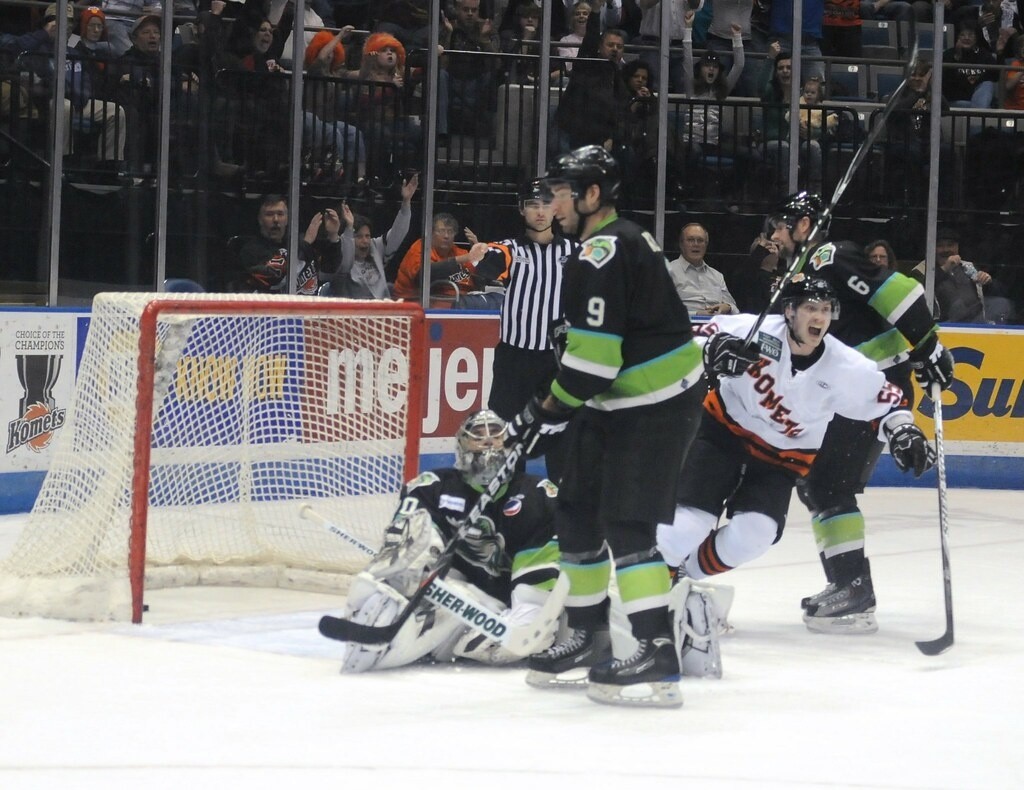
[338,409,732,681]
[503,144,709,705]
[0,0,1024,212]
[233,174,506,310]
[768,191,954,635]
[913,229,995,323]
[864,240,895,269]
[478,177,578,422]
[670,222,740,315]
[656,279,936,585]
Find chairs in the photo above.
[495,17,1024,214]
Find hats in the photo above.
[695,51,726,71]
[955,21,981,39]
[937,227,959,239]
[130,15,163,36]
[80,7,105,33]
[364,33,406,68]
[44,3,74,18]
[306,32,344,68]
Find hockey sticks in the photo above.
[742,33,921,349]
[911,377,958,657]
[317,444,526,645]
[294,503,571,657]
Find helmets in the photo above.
[453,409,507,477]
[517,177,553,210]
[770,190,832,232]
[538,144,621,205]
[783,273,840,321]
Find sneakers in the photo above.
[667,554,694,588]
[802,546,864,610]
[588,627,683,709]
[804,550,876,634]
[676,590,722,675]
[528,609,613,689]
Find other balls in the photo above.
[142,604,150,612]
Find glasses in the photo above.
[433,230,457,236]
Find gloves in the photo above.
[547,319,568,372]
[502,397,570,463]
[910,337,955,396]
[883,419,937,478]
[702,331,760,387]
